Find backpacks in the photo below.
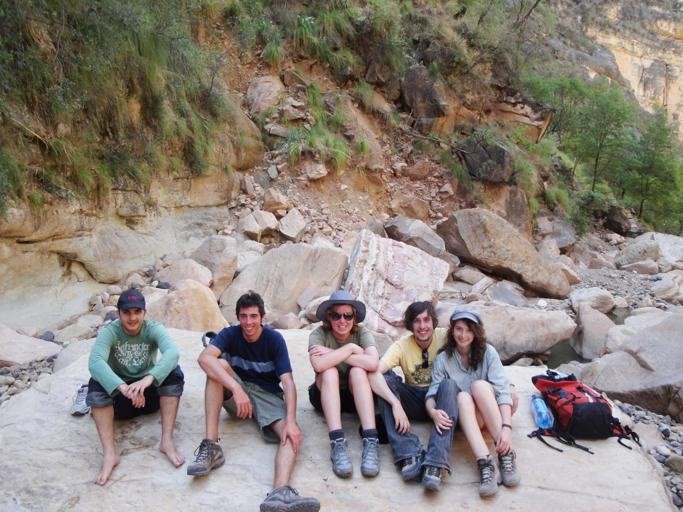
[531,370,613,438]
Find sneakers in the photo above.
[260,485,320,511]
[330,438,353,478]
[362,438,380,475]
[71,384,90,416]
[499,451,520,486]
[187,439,225,476]
[476,454,501,498]
[402,448,426,480]
[424,465,442,492]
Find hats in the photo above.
[316,291,365,323]
[117,290,145,311]
[450,309,483,324]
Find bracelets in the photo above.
[502,424,512,428]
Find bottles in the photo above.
[530,392,554,430]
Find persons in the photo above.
[308,290,381,477]
[367,300,458,492]
[85,289,185,485]
[187,291,321,512]
[424,310,520,498]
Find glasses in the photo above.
[422,349,428,369]
[329,312,354,322]
